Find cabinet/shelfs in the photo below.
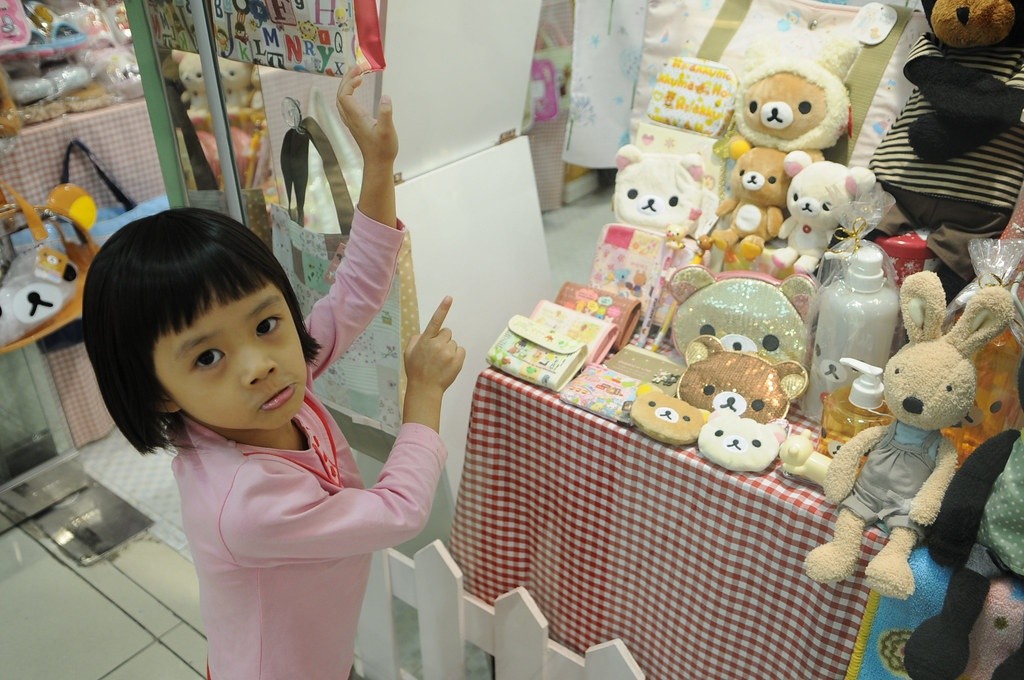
[124,1,550,462]
[2,256,88,525]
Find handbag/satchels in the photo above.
[6,140,170,355]
[268,117,422,439]
[0,174,99,353]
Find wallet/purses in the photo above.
[487,56,813,472]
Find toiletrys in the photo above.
[801,241,902,430]
[813,357,898,472]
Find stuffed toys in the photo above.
[734,21,860,162]
[615,144,705,237]
[217,57,251,107]
[804,266,1012,601]
[856,0,1024,306]
[171,50,208,110]
[778,150,877,275]
[904,351,1024,680]
[712,136,792,261]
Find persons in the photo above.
[79,65,466,680]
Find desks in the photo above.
[0,95,169,448]
[450,370,1024,679]
